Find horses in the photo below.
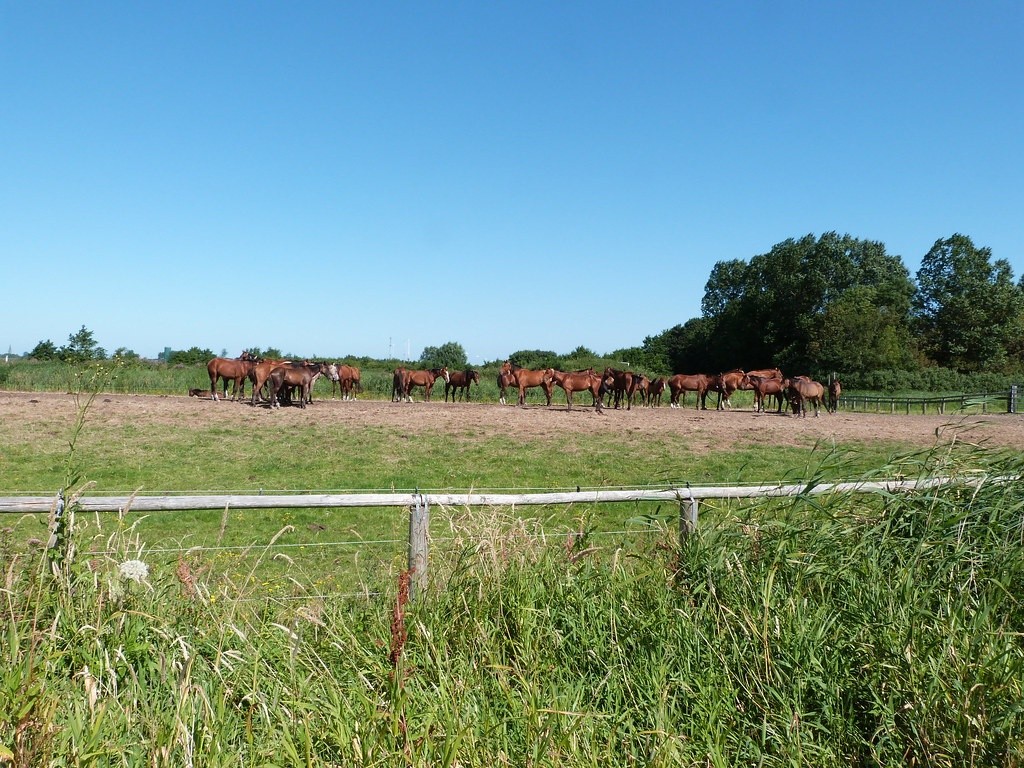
[186,350,844,420]
